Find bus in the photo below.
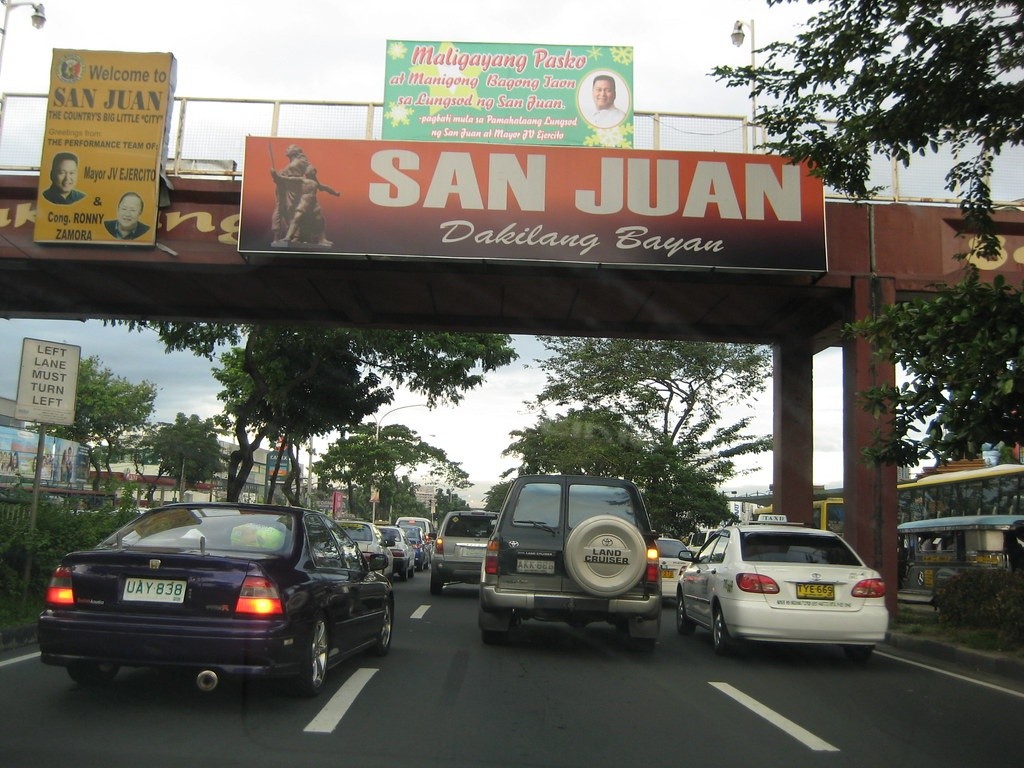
[896,514,1024,596]
[896,464,1024,524]
[740,495,844,539]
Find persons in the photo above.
[104,193,150,239]
[0,446,74,483]
[268,143,341,246]
[587,75,626,125]
[43,152,86,205]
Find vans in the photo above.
[397,516,437,554]
[682,528,730,559]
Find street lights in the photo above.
[368,404,428,522]
[732,18,758,155]
[0,2,48,90]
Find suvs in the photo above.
[477,474,663,650]
[429,509,502,598]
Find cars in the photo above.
[399,523,434,572]
[675,515,889,660]
[371,525,417,582]
[306,518,396,585]
[37,500,395,699]
[652,538,695,599]
